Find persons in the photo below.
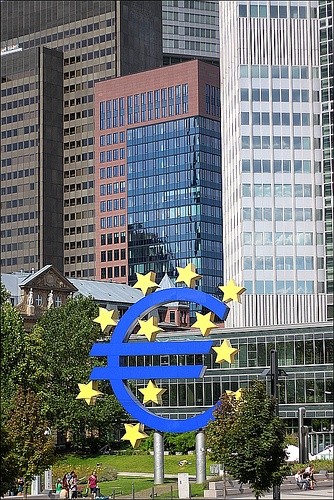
[87,471,98,500]
[55,471,78,500]
[18,481,24,494]
[295,464,317,486]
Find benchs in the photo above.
[225,473,333,494]
